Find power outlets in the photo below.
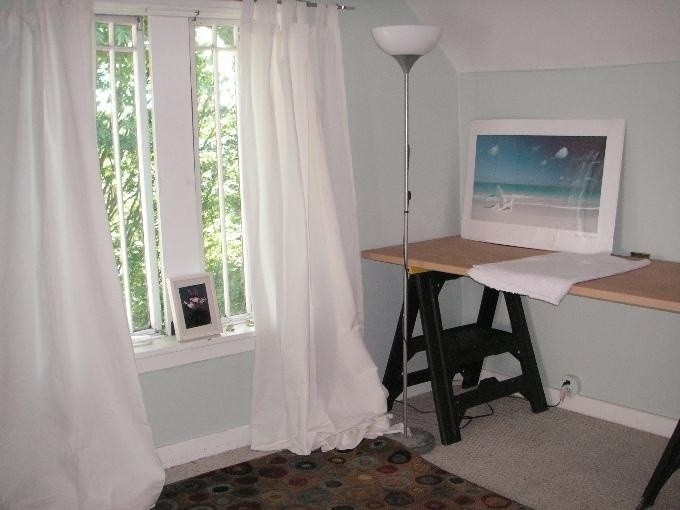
[560,376,581,397]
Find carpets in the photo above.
[139,438,532,509]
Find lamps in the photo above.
[373,25,441,456]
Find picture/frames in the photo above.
[163,270,221,344]
[460,118,626,256]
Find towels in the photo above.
[465,254,651,307]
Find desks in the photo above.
[362,234,680,510]
